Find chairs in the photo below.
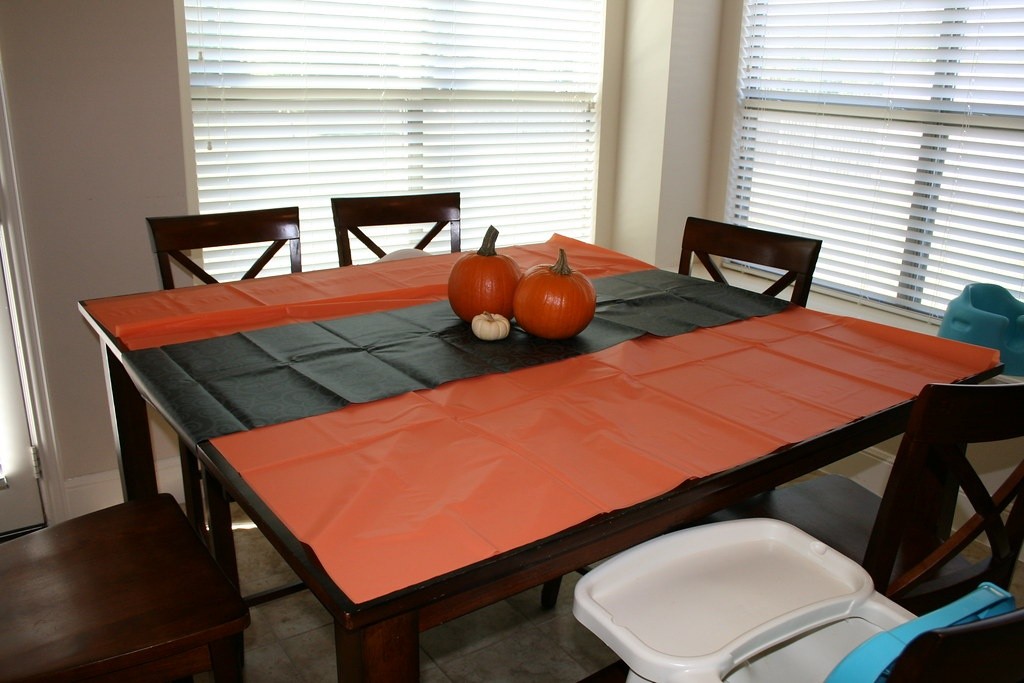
[146,206,304,629]
[678,216,821,309]
[0,491,246,683]
[331,191,461,266]
[693,383,1024,618]
[821,580,1015,683]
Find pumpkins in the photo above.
[472,311,510,340]
[448,224,523,323]
[512,247,597,338]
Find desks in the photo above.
[77,234,1004,683]
[571,518,918,683]
